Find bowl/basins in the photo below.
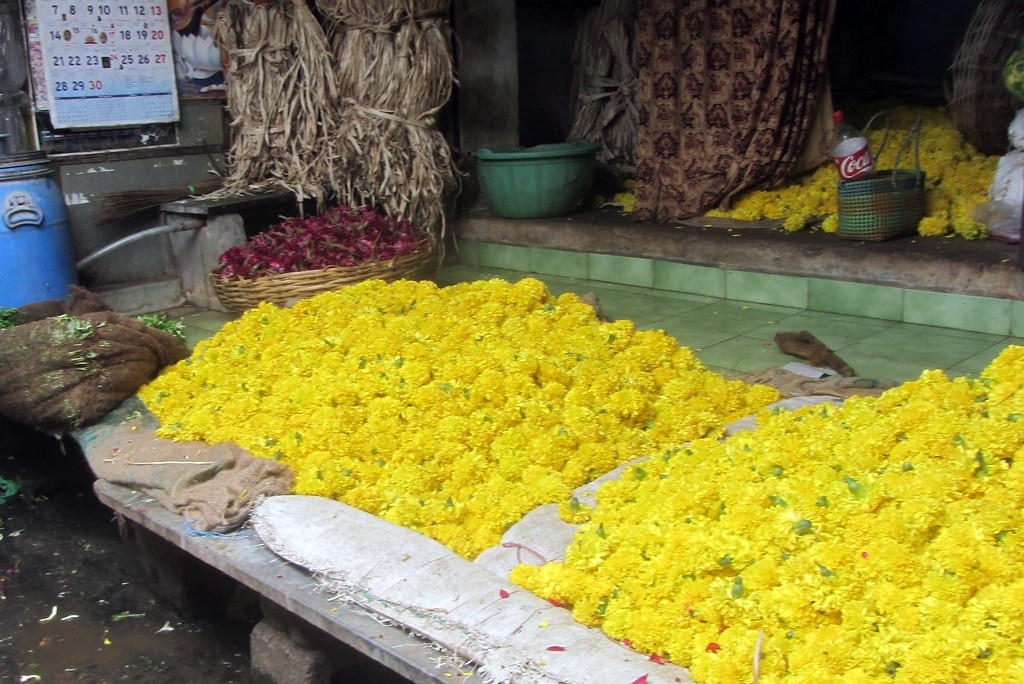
[473,142,600,219]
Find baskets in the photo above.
[207,242,439,319]
[837,112,926,242]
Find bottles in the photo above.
[830,111,877,184]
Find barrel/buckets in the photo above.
[0,151,79,312]
